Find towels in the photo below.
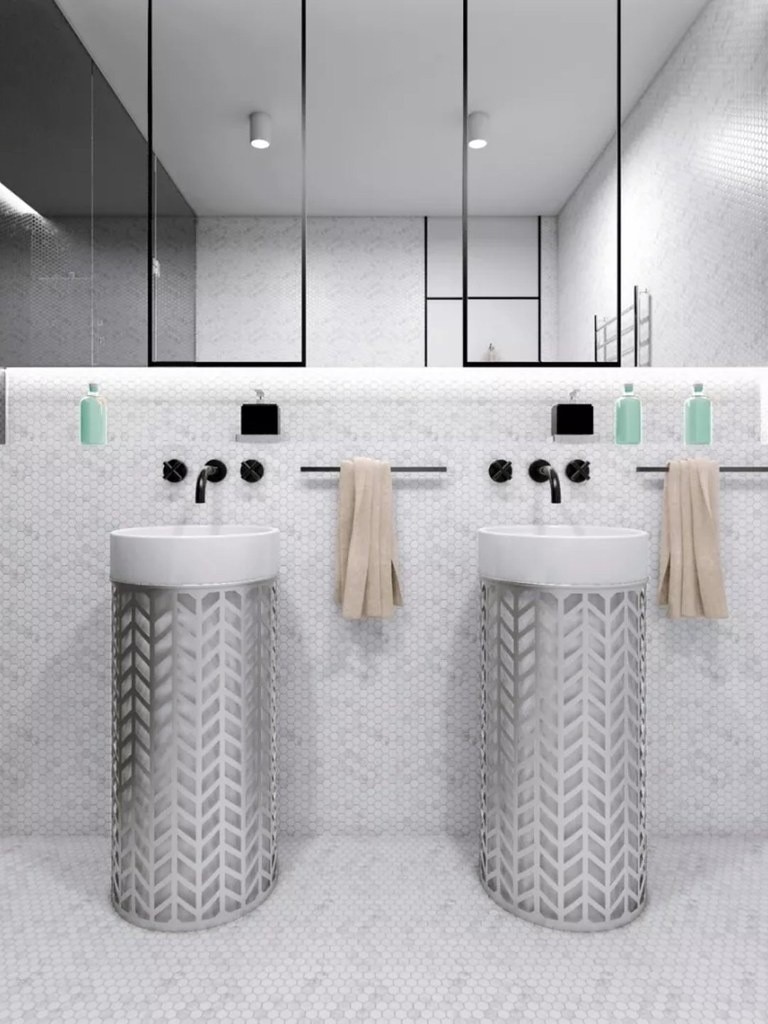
[336,456,405,620]
[658,458,729,619]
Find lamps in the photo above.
[249,111,272,149]
[468,113,487,149]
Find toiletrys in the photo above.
[80,381,108,446]
[683,383,713,445]
[614,382,642,445]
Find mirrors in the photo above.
[0,0,768,369]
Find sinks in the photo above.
[479,524,656,589]
[109,523,281,587]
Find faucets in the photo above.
[194,465,216,505]
[537,466,563,505]
